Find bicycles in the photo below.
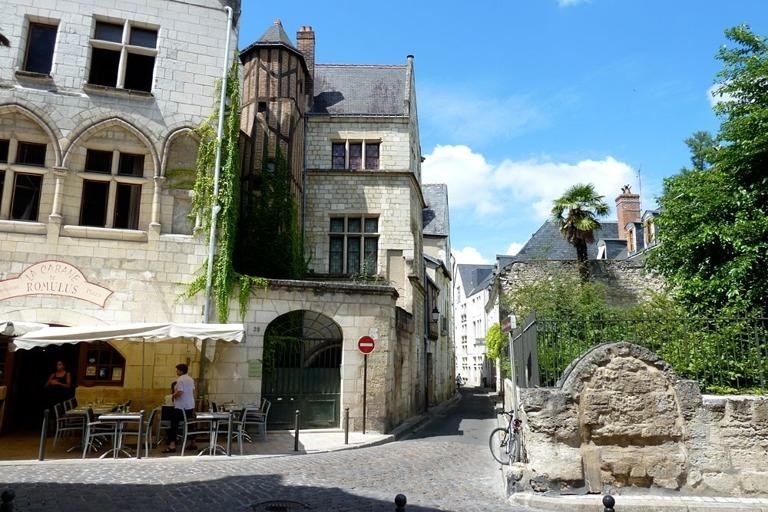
[489,410,522,465]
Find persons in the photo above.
[161,364,198,453]
[45,360,72,401]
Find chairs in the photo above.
[53,398,274,459]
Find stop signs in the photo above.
[357,336,375,355]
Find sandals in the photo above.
[162,447,176,453]
